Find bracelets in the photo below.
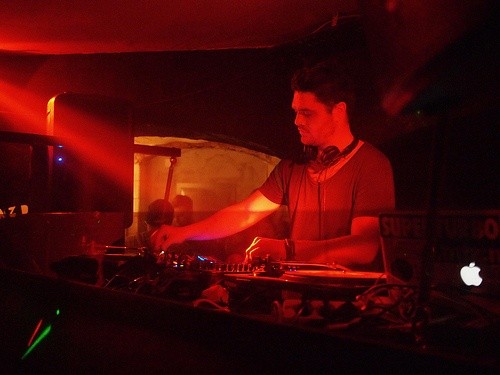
[285,239,295,261]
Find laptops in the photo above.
[377,208,500,300]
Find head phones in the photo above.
[301,133,360,167]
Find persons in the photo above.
[148,65,394,264]
